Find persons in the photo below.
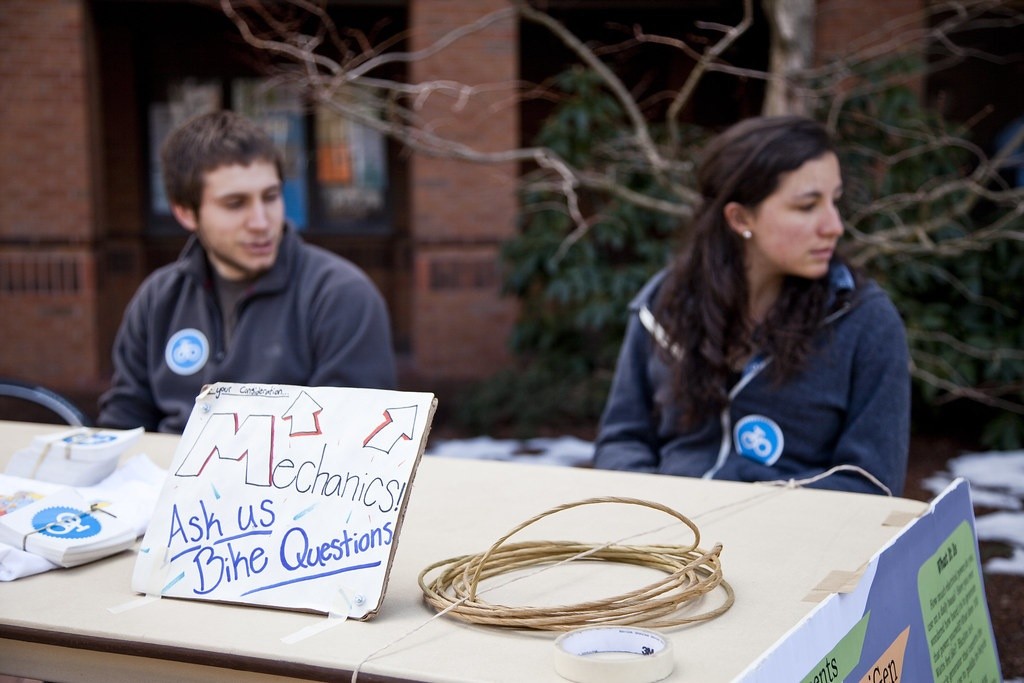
[98,108,395,439]
[588,114,910,497]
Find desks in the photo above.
[0,417,932,683]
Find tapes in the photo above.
[552,625,676,683]
